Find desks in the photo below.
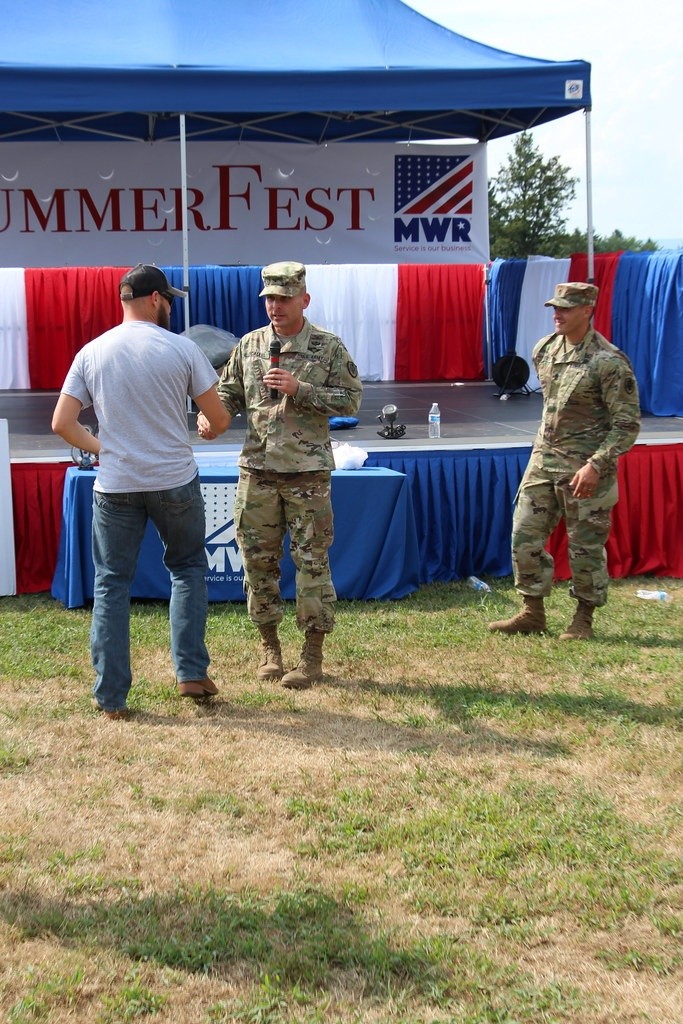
[51,465,422,609]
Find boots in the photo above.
[488,595,548,636]
[281,630,324,688]
[559,600,595,642]
[257,621,283,680]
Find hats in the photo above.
[259,261,306,297]
[119,263,187,300]
[544,282,599,308]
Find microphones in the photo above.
[269,340,281,399]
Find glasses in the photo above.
[158,290,174,306]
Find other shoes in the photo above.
[179,675,219,697]
[91,698,129,720]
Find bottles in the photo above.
[428,403,441,438]
[467,576,489,591]
[638,591,667,600]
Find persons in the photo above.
[50,263,230,716]
[199,261,362,689]
[490,282,641,642]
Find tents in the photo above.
[0,1,631,414]
